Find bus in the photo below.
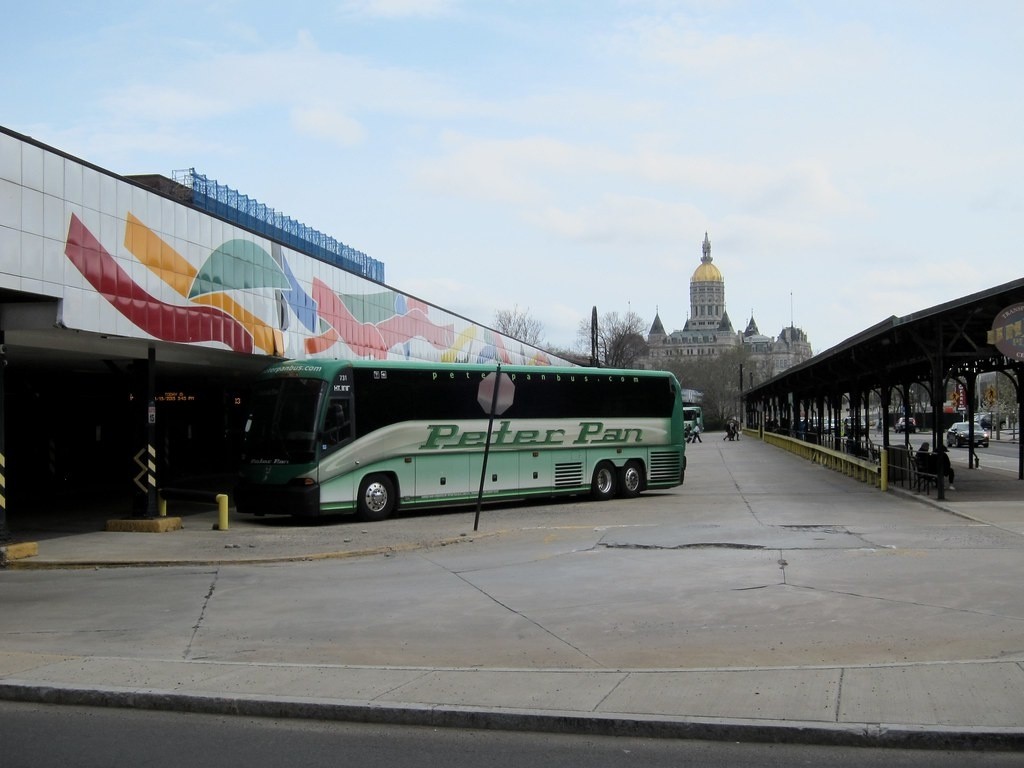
[216,357,688,525]
[682,406,704,437]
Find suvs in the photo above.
[894,417,916,433]
[843,417,866,437]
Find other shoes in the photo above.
[948,486,955,490]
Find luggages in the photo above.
[686,437,692,443]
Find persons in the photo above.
[767,417,792,431]
[723,420,741,441]
[684,424,692,443]
[692,424,702,443]
[843,418,850,436]
[915,442,956,490]
[845,436,879,460]
[799,419,818,444]
[875,418,882,437]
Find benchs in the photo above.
[910,457,940,488]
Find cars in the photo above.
[973,412,1006,430]
[792,417,835,434]
[947,421,989,448]
